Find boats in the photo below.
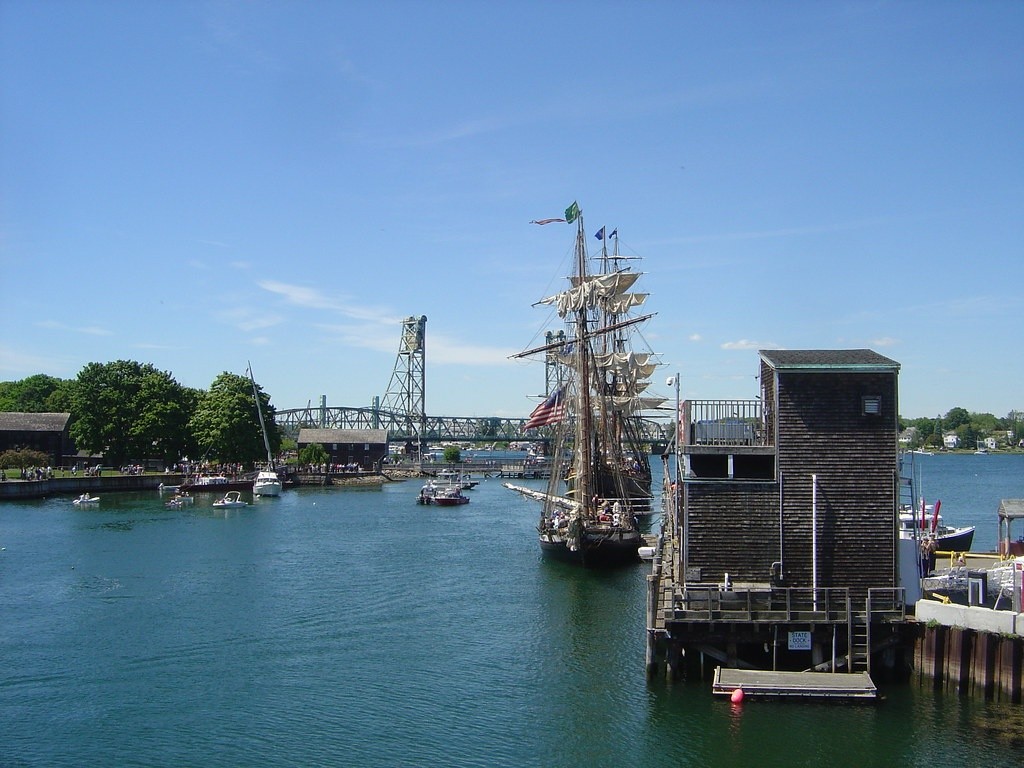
[650,347,927,707]
[253,471,284,499]
[212,491,249,509]
[71,494,101,504]
[899,502,977,552]
[156,483,194,510]
[393,442,544,465]
[415,464,481,508]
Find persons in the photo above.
[21,463,102,481]
[920,532,939,578]
[310,460,360,473]
[174,488,189,497]
[165,460,244,481]
[79,492,91,500]
[546,509,567,528]
[119,463,145,474]
[598,499,623,521]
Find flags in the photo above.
[595,227,604,240]
[565,201,580,224]
[523,385,567,432]
[609,227,618,239]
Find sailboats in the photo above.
[500,202,680,556]
